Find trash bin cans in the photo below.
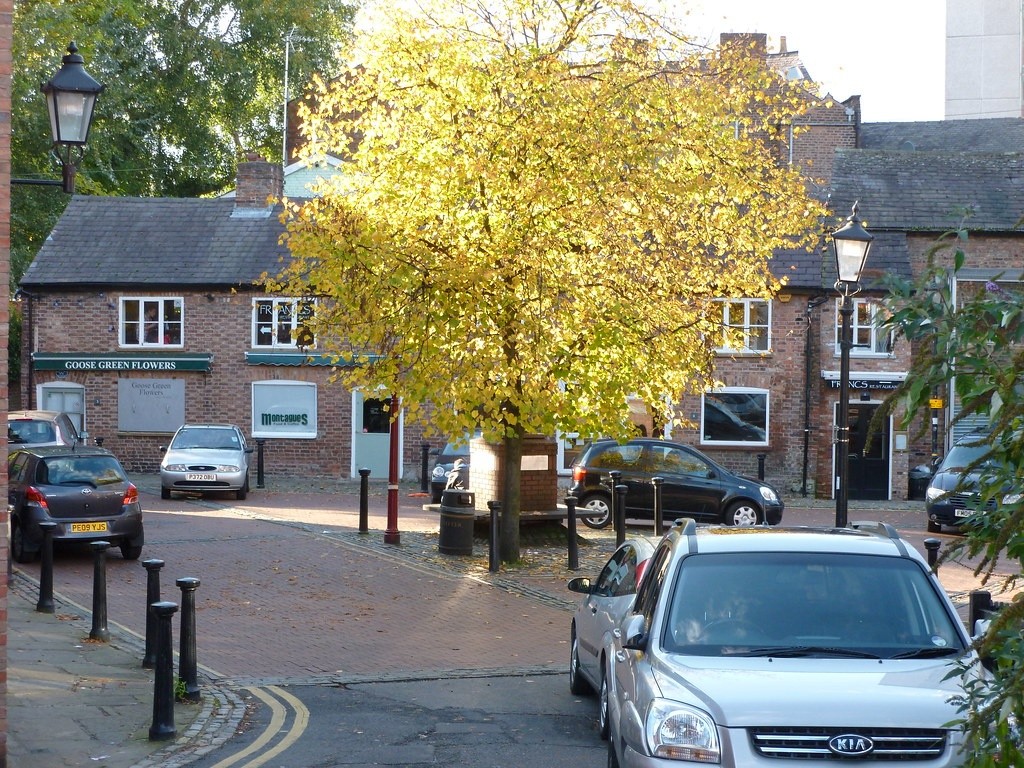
[439,490,476,555]
[908,469,932,501]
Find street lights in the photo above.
[829,202,873,527]
[923,276,943,468]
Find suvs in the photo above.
[602,517,1020,768]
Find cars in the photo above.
[158,423,254,500]
[564,438,784,529]
[704,393,765,439]
[8,410,82,452]
[926,433,1024,533]
[429,428,482,503]
[567,534,664,698]
[8,446,144,565]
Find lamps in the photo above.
[10,40,106,194]
